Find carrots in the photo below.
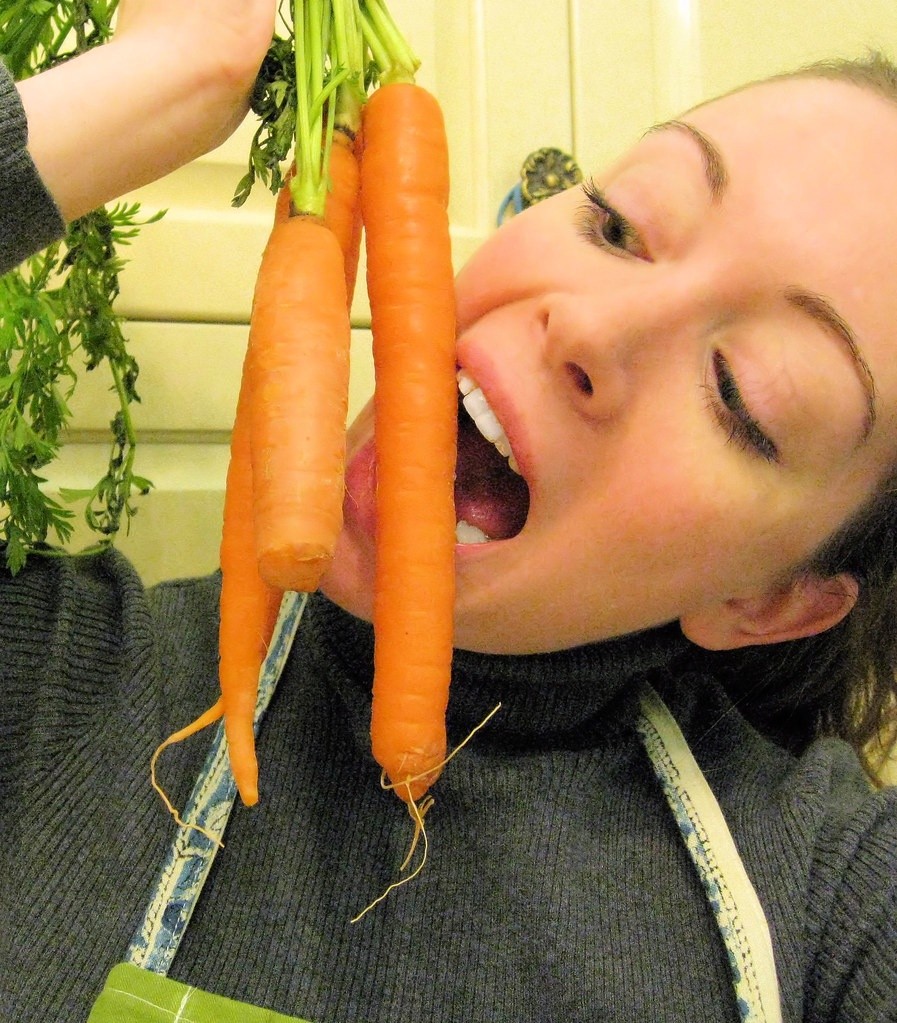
[150,83,459,828]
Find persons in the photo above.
[0,1,897,1023]
